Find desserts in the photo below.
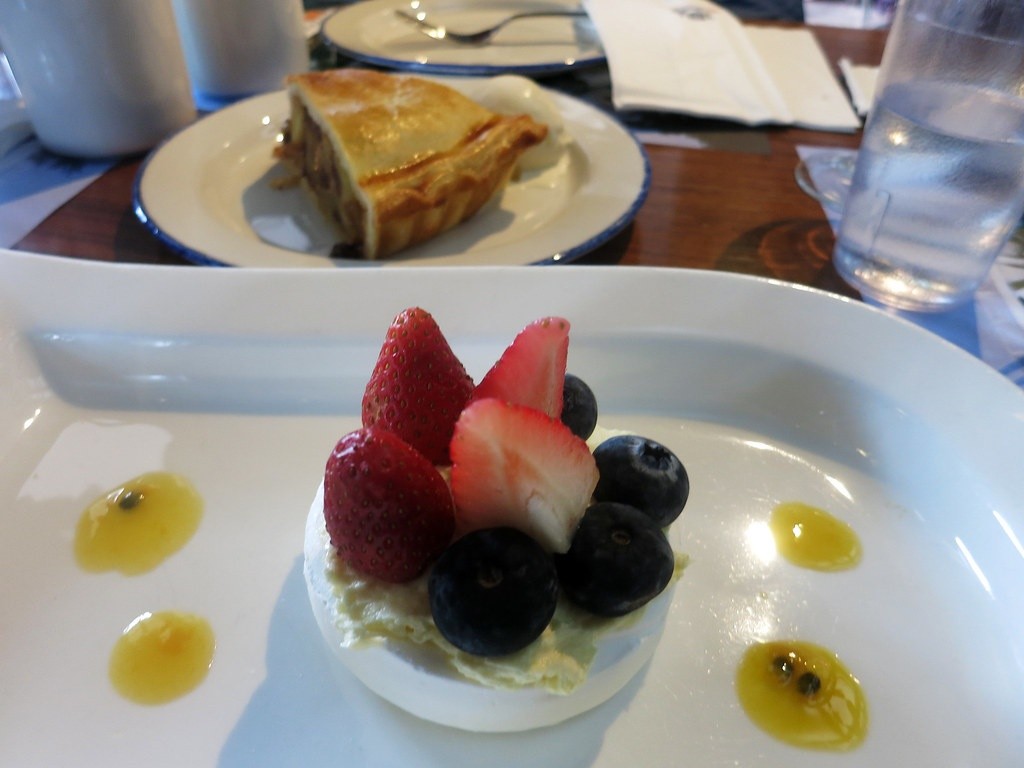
[305,306,690,733]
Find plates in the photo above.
[1,247,1022,767]
[320,0,607,77]
[130,73,650,265]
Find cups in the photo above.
[832,1,1024,312]
[0,1,197,159]
[173,0,311,110]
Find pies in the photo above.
[273,67,550,259]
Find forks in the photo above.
[394,7,587,43]
